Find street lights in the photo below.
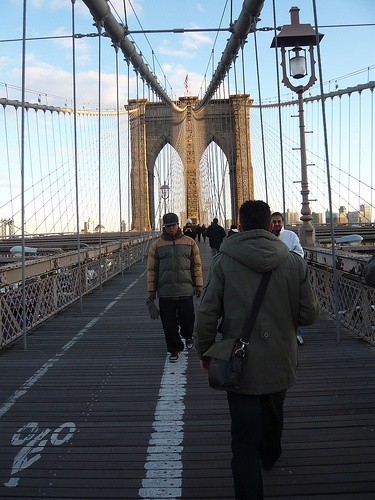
[160,181,170,215]
[270,5,325,248]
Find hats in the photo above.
[162,213,178,226]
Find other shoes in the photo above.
[185,337,194,349]
[168,351,182,362]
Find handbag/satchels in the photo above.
[203,338,248,391]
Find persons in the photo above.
[190,200,322,500]
[182,218,207,243]
[147,213,203,362]
[228,225,239,238]
[206,219,226,257]
[270,213,306,345]
